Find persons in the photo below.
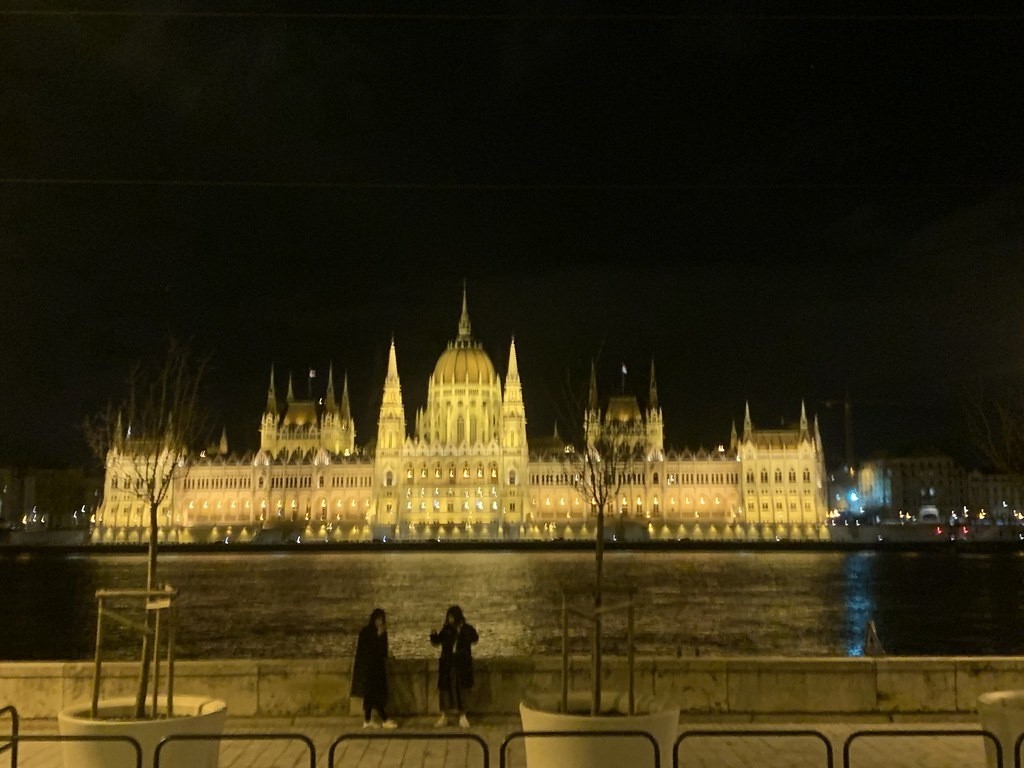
[430,605,479,727]
[350,609,398,728]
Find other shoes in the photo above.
[364,720,381,729]
[383,719,399,728]
[434,714,449,726]
[458,714,470,728]
